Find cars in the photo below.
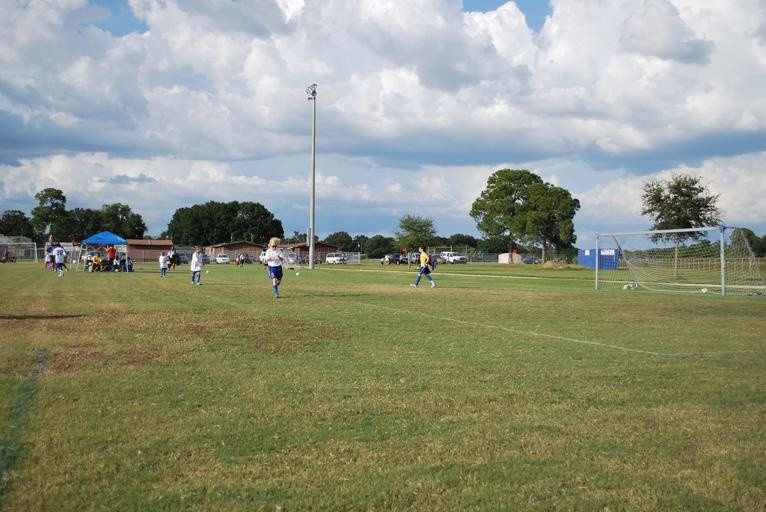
[380,250,468,265]
[214,253,230,264]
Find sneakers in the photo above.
[45,269,69,277]
[409,284,417,289]
[431,282,435,288]
[159,274,281,298]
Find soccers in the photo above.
[206,270,210,274]
[701,287,709,294]
[296,272,300,277]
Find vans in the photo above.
[259,247,298,264]
[175,249,210,265]
[325,252,348,264]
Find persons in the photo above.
[190,247,204,286]
[88,251,103,272]
[157,251,168,278]
[235,255,240,268]
[124,256,132,272]
[44,242,70,278]
[264,237,285,299]
[239,251,246,267]
[111,254,121,272]
[406,245,437,288]
[166,246,176,271]
[103,244,116,264]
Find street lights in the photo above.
[304,83,318,271]
